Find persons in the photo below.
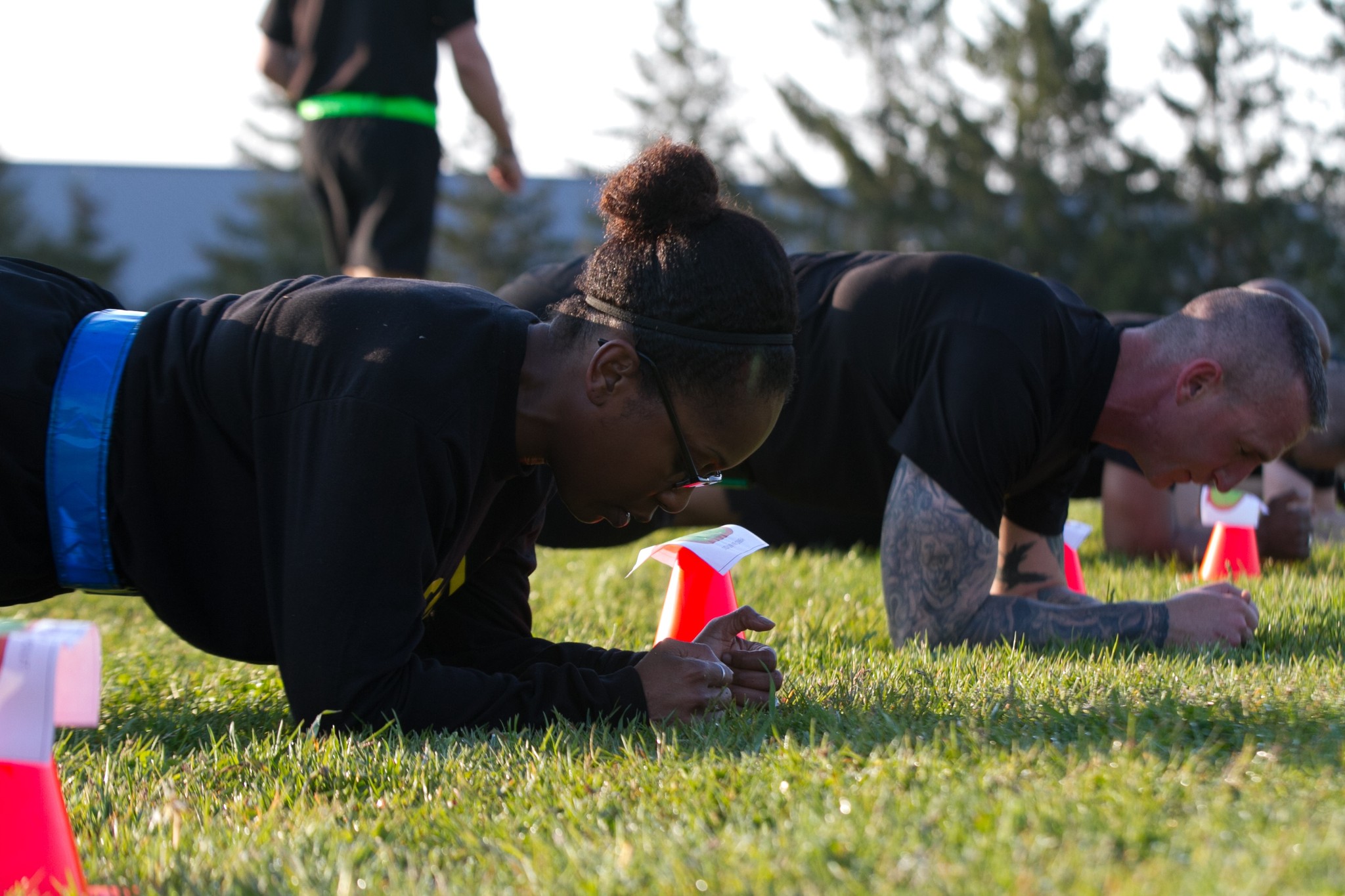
[0,146,804,739]
[492,250,1344,661]
[255,2,528,283]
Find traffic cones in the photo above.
[654,547,745,646]
[0,632,87,896]
[1200,522,1259,583]
[1064,542,1085,593]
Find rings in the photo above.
[713,662,726,686]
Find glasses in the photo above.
[597,338,724,491]
